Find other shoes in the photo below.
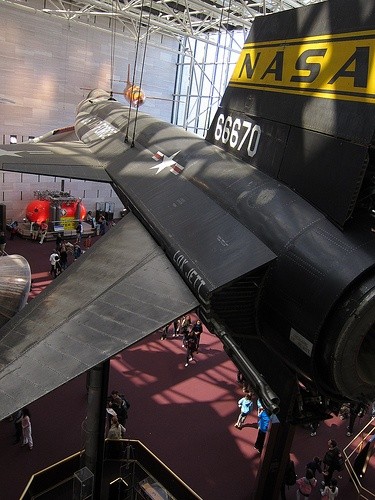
[346,432,353,436]
[172,334,175,337]
[185,363,188,367]
[29,446,33,450]
[189,357,193,362]
[161,337,163,340]
[234,421,242,428]
[12,441,18,444]
[177,334,179,337]
[310,432,316,437]
[20,444,26,447]
[346,426,350,430]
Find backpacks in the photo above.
[336,452,344,472]
[118,393,130,410]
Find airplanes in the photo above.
[0,1,375,428]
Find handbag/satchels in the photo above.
[321,489,330,500]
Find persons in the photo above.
[308,403,364,437]
[104,391,129,440]
[83,235,93,250]
[31,221,49,245]
[9,407,33,451]
[10,221,18,240]
[235,370,270,453]
[85,209,114,235]
[0,232,7,255]
[49,234,82,279]
[296,439,340,500]
[74,222,82,245]
[157,314,203,367]
